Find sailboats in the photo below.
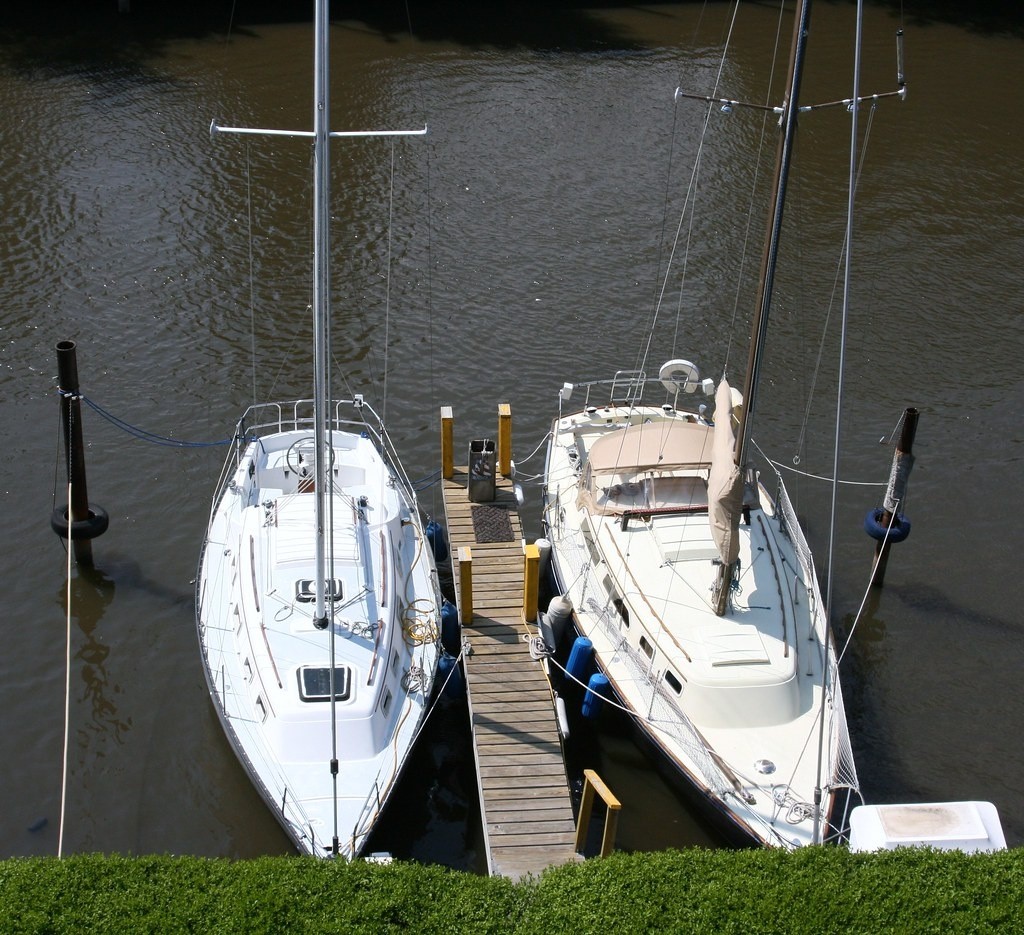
[545,0,909,850]
[191,0,446,863]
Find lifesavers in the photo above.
[659,358,699,394]
